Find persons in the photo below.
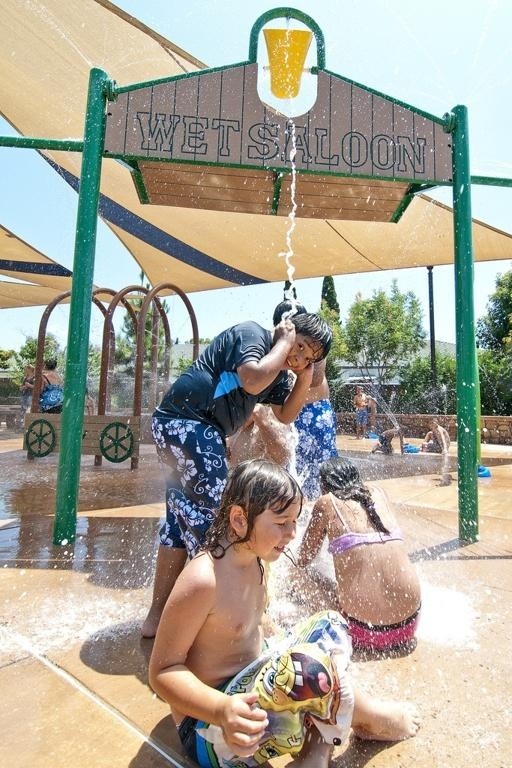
[296,457,422,653]
[418,420,450,453]
[226,421,265,473]
[85,394,96,409]
[371,427,408,456]
[225,404,298,467]
[273,299,336,521]
[16,363,35,434]
[39,358,65,414]
[148,459,421,767]
[139,314,334,639]
[350,386,371,439]
